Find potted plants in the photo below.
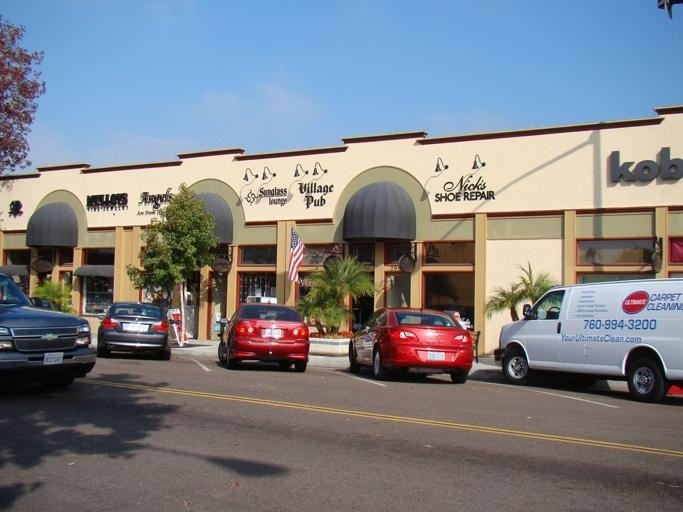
[297,255,381,356]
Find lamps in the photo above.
[294,164,308,177]
[244,169,258,181]
[313,162,327,175]
[472,155,485,169]
[435,157,448,172]
[262,167,276,180]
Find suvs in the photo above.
[0,271,96,390]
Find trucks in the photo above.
[494,275,682,403]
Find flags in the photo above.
[286,228,305,283]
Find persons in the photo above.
[443,311,468,331]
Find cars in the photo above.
[11,297,65,315]
[216,304,310,373]
[95,300,175,361]
[347,305,471,385]
